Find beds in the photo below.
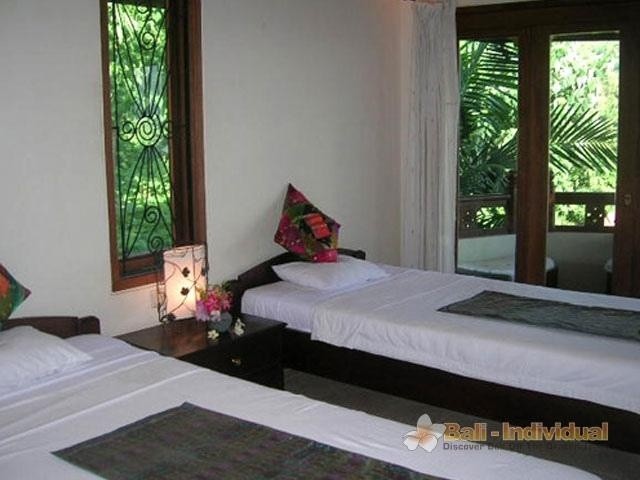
[0,316,601,480]
[224,248,640,456]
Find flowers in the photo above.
[193,279,236,322]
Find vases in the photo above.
[209,339,219,346]
[208,329,220,341]
[208,311,234,335]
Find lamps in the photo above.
[152,239,209,324]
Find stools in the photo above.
[457,254,558,288]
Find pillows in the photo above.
[271,182,391,295]
[0,325,94,395]
[0,263,32,331]
